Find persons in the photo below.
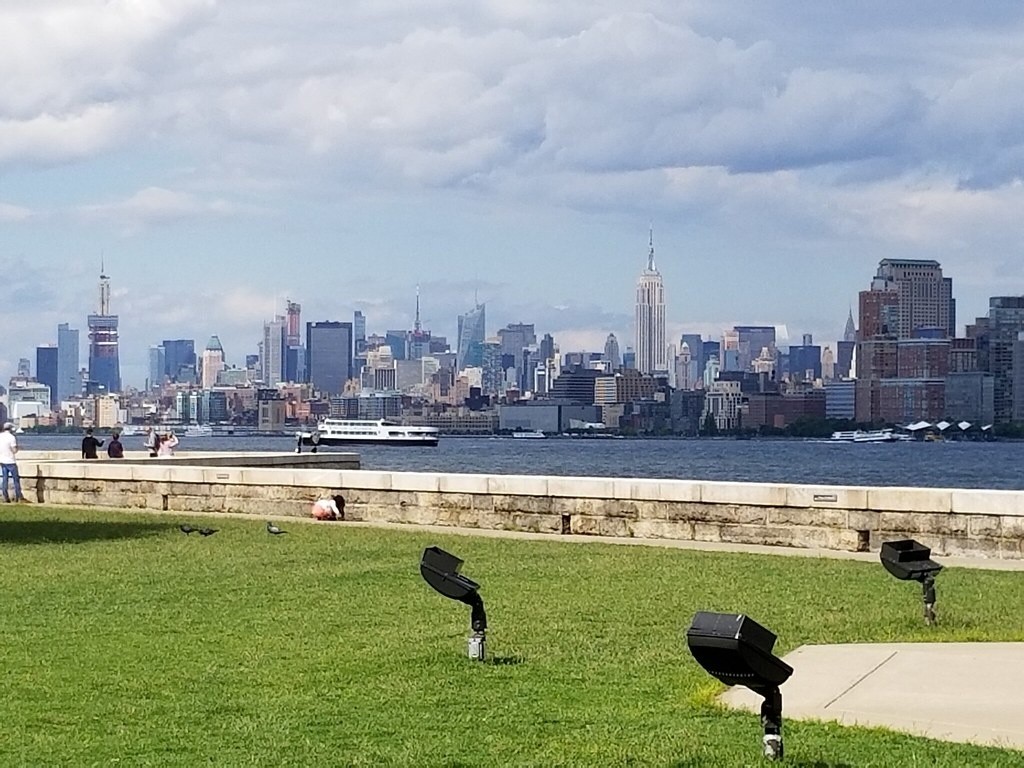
[143,427,179,458]
[108,433,124,458]
[82,429,106,459]
[312,495,345,522]
[0,422,32,503]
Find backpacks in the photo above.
[153,433,160,450]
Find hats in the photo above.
[3,422,16,429]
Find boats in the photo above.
[294,418,440,447]
[512,432,547,439]
[830,427,937,443]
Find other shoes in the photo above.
[16,498,32,503]
[4,500,10,503]
